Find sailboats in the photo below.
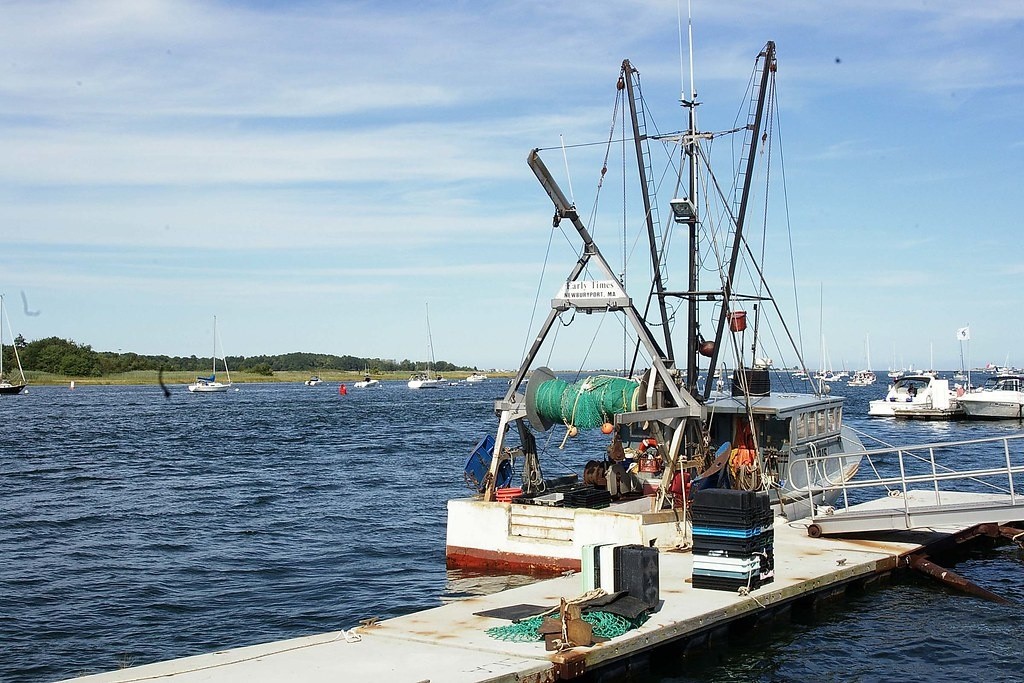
[406,364,438,389]
[188,314,233,393]
[0,293,30,395]
[423,301,447,384]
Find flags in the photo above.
[957,326,969,341]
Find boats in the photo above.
[465,373,489,382]
[507,378,529,386]
[304,371,324,387]
[712,283,1024,423]
[446,378,472,387]
[354,363,380,389]
[434,0,869,579]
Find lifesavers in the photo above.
[638,438,660,454]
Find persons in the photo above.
[908,383,914,393]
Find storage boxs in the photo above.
[733,370,771,397]
[691,488,774,592]
[495,482,612,508]
[580,542,659,609]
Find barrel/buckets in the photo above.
[727,311,746,332]
[496,488,523,503]
